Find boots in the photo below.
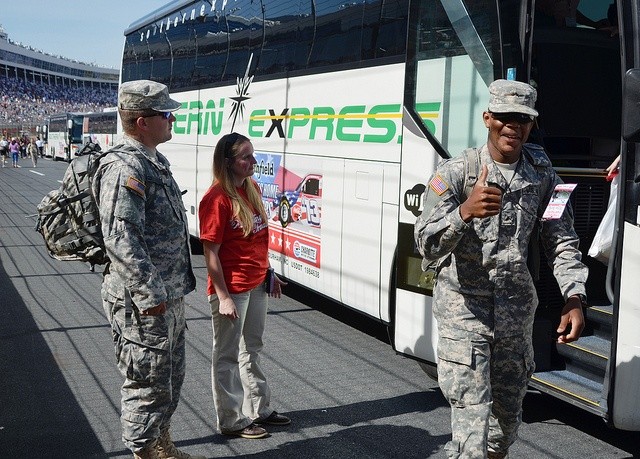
[160,427,206,459]
[132,440,164,459]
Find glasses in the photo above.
[139,112,174,120]
[490,112,536,123]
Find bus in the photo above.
[82,106,117,154]
[47,111,81,162]
[118,2,640,430]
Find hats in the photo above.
[487,79,539,116]
[118,79,182,113]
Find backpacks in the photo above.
[35,147,156,272]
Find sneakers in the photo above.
[256,410,291,426]
[221,420,268,438]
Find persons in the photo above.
[35,137,43,159]
[413,79,590,458]
[9,140,20,168]
[89,78,196,458]
[56,81,118,114]
[26,138,40,168]
[15,135,29,159]
[0,136,8,168]
[0,75,56,124]
[200,132,288,438]
